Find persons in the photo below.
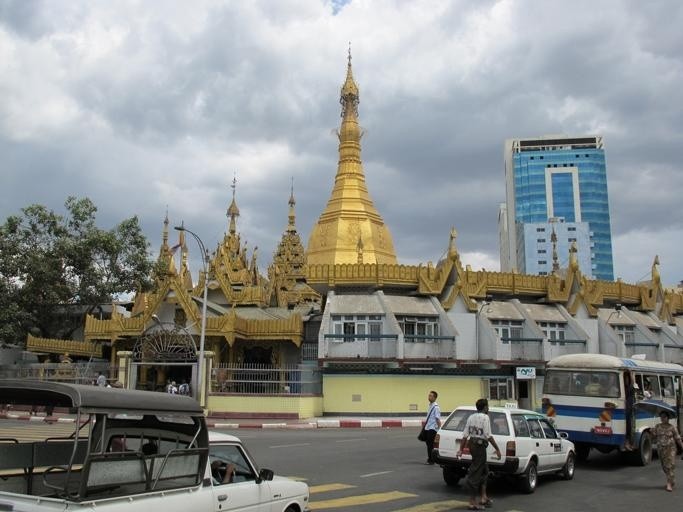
[142,442,158,456]
[456,397,501,510]
[186,444,234,485]
[91,371,113,389]
[422,390,440,465]
[649,411,683,491]
[552,371,680,400]
[165,377,191,395]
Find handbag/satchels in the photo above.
[418,430,426,441]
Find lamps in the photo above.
[604,310,623,325]
[476,303,493,318]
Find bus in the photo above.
[540,353,683,466]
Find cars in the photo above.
[432,405,576,495]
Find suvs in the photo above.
[0,376,312,512]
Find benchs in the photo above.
[0,438,209,502]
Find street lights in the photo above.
[173,226,210,402]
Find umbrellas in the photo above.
[633,397,677,439]
